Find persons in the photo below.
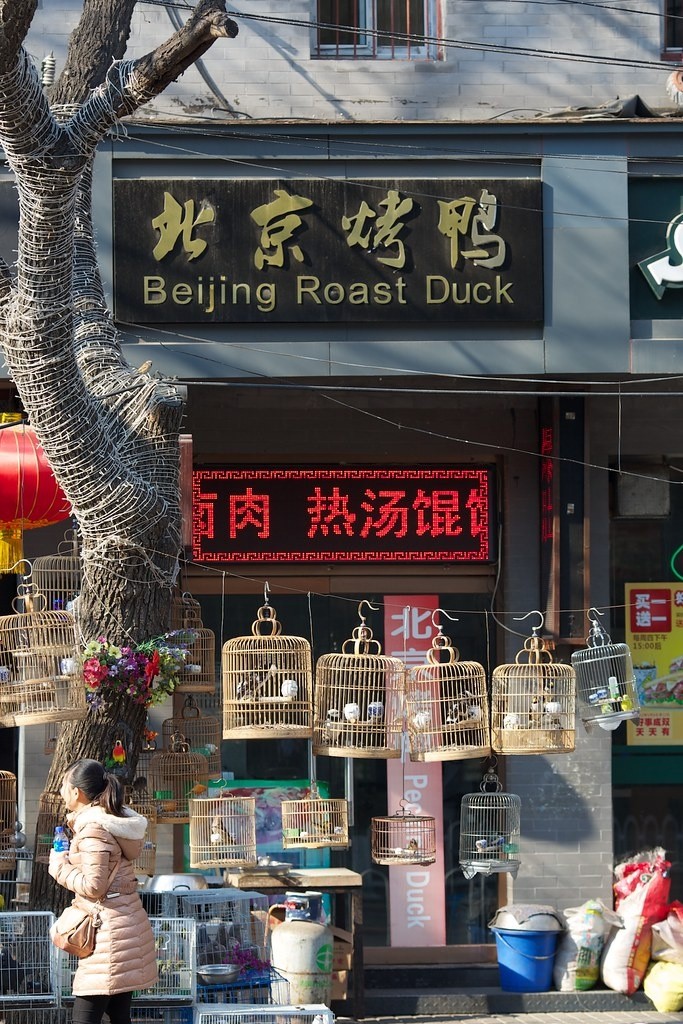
[47,756,160,1024]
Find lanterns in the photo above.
[0,412,74,571]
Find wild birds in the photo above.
[599,698,614,714]
[533,682,555,702]
[409,839,419,853]
[530,697,543,723]
[131,775,147,795]
[311,820,331,831]
[185,785,207,797]
[211,816,237,846]
[446,694,467,724]
[621,694,633,711]
[152,923,188,972]
[167,633,202,645]
[322,709,344,743]
[281,679,298,699]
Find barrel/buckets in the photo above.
[490,925,561,993]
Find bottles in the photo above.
[53,827,70,862]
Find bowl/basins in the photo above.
[495,911,565,932]
[144,873,210,894]
[196,964,242,985]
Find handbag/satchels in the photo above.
[49,907,102,959]
